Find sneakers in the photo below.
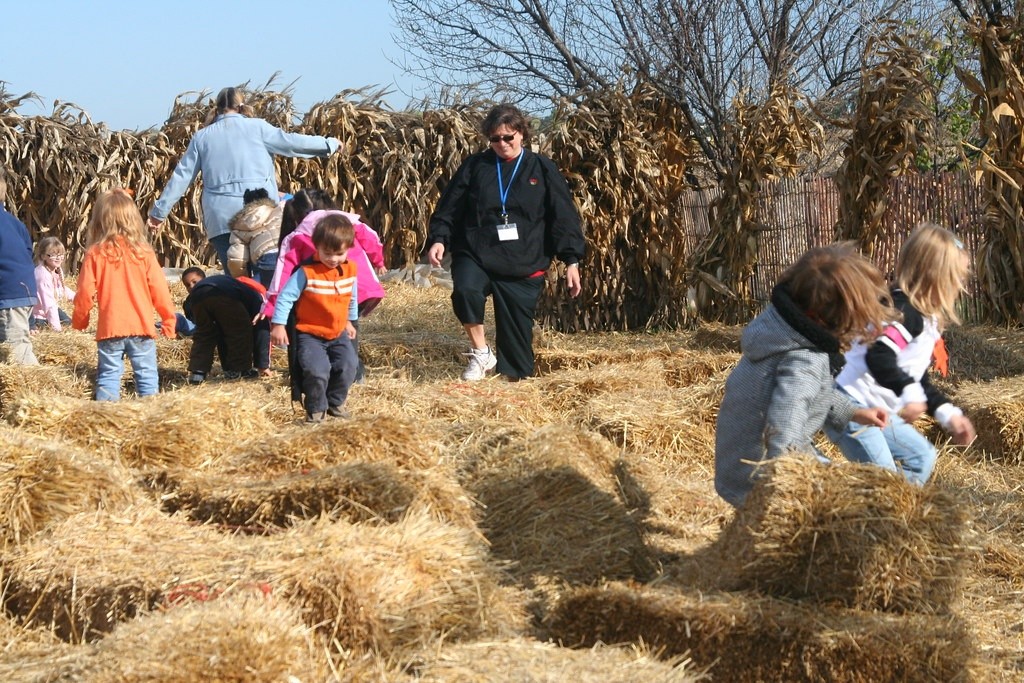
[461,344,497,380]
[327,405,350,418]
[306,410,327,423]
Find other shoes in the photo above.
[191,370,207,384]
[225,367,259,379]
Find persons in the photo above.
[147,87,344,273]
[0,175,39,366]
[271,214,359,427]
[28,238,76,331]
[427,104,586,380]
[226,188,287,348]
[155,268,271,384]
[72,189,177,403]
[715,241,889,510]
[822,222,975,487]
[252,188,387,383]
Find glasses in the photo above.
[489,129,518,142]
[46,253,65,259]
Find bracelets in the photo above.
[572,262,579,269]
